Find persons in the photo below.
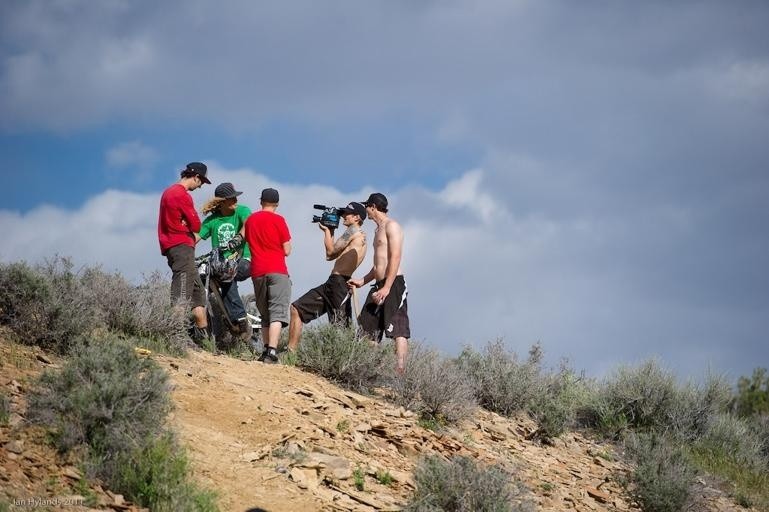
[244,187,292,363]
[157,161,212,346]
[275,202,368,357]
[194,182,253,343]
[345,193,411,377]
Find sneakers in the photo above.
[257,351,281,364]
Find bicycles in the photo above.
[194,246,263,356]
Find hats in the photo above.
[359,193,388,210]
[214,183,243,200]
[186,162,211,185]
[339,202,366,220]
[258,188,279,203]
